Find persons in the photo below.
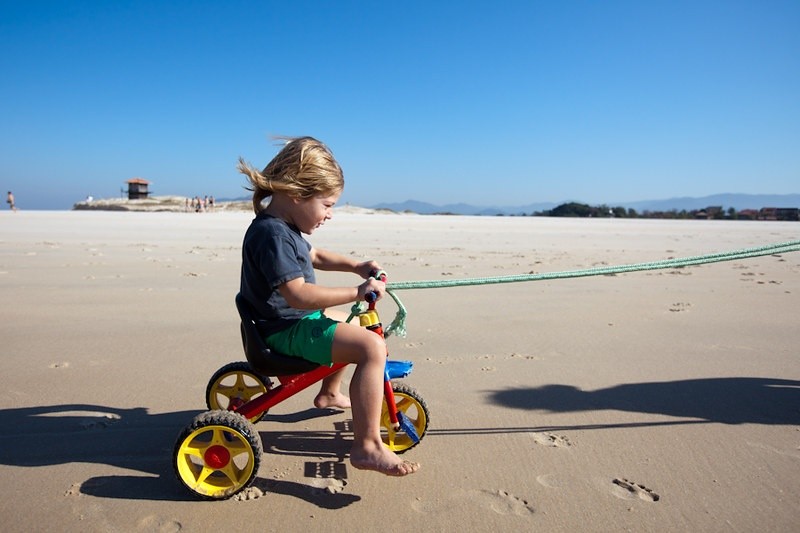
[7,191,16,212]
[234,136,421,477]
[184,195,215,213]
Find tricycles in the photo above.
[174,267,430,500]
